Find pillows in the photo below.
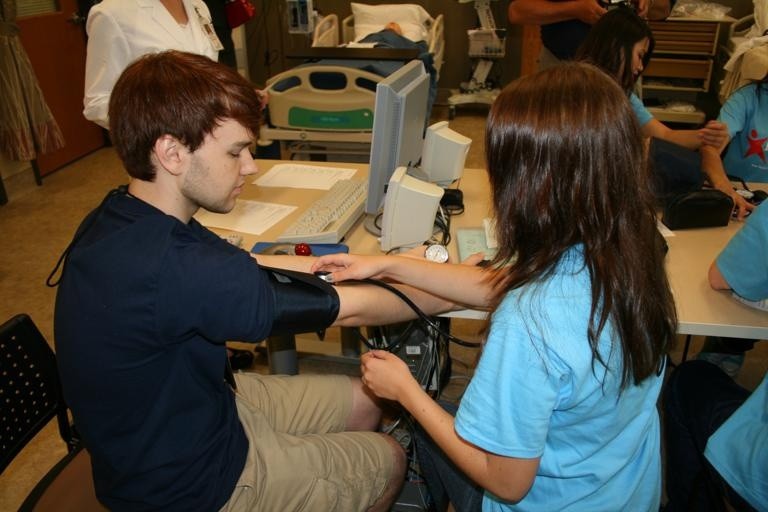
[349,1,434,38]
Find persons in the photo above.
[42,46,495,512]
[79,0,227,142]
[272,18,427,95]
[657,194,767,510]
[505,0,680,77]
[694,69,767,379]
[306,64,682,512]
[571,7,730,159]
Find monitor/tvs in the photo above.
[363,59,443,237]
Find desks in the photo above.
[189,158,767,383]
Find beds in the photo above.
[246,1,448,167]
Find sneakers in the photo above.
[697,350,745,380]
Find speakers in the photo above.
[420,120,470,181]
[379,165,445,252]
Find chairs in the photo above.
[0,312,110,511]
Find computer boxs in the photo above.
[366,317,450,409]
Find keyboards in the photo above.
[276,179,366,244]
